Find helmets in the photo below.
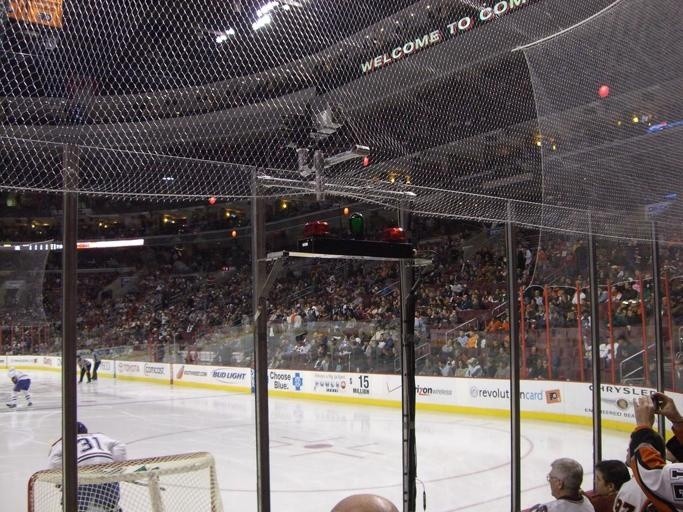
[77,422,88,433]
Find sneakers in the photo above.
[28,402,32,406]
[91,377,97,380]
[9,404,16,408]
[78,380,91,384]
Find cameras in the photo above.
[650,396,664,412]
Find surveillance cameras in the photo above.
[309,107,345,142]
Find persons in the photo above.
[2,211,681,392]
[528,392,683,512]
[330,493,400,512]
[44,420,128,512]
[7,364,35,409]
